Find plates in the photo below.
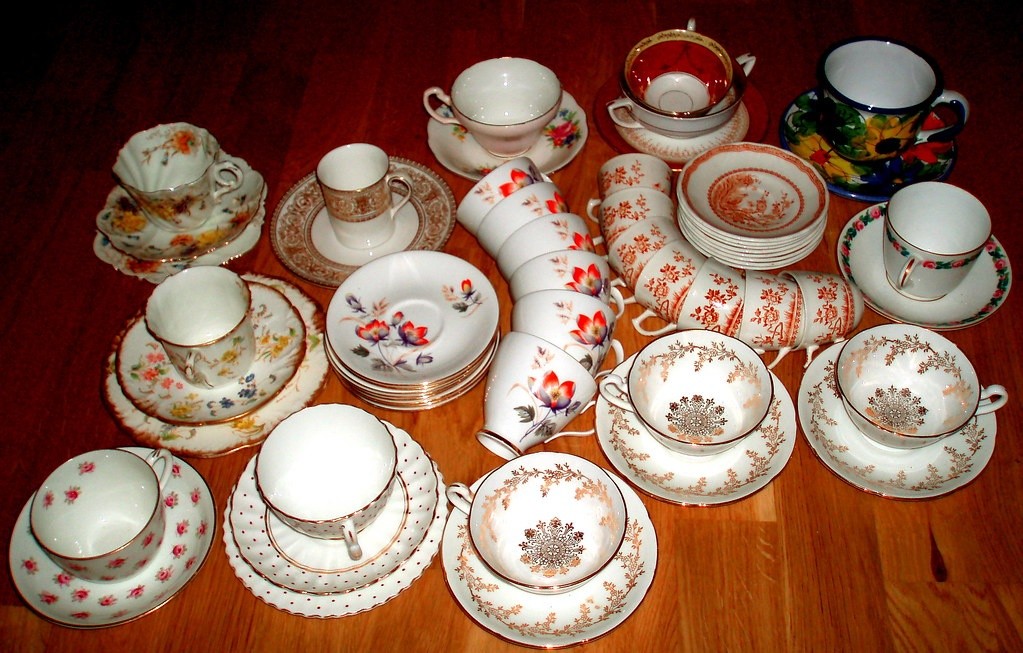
[9,446,218,629]
[839,197,1011,332]
[102,273,332,458]
[222,421,448,618]
[96,150,264,262]
[273,157,501,411]
[783,85,960,201]
[594,77,769,169]
[93,184,267,284]
[594,353,800,507]
[428,90,588,183]
[797,346,998,498]
[115,280,306,425]
[443,470,658,649]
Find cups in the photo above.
[30,449,173,581]
[111,122,244,233]
[424,18,1009,595]
[255,402,397,559]
[316,144,411,252]
[145,267,256,390]
